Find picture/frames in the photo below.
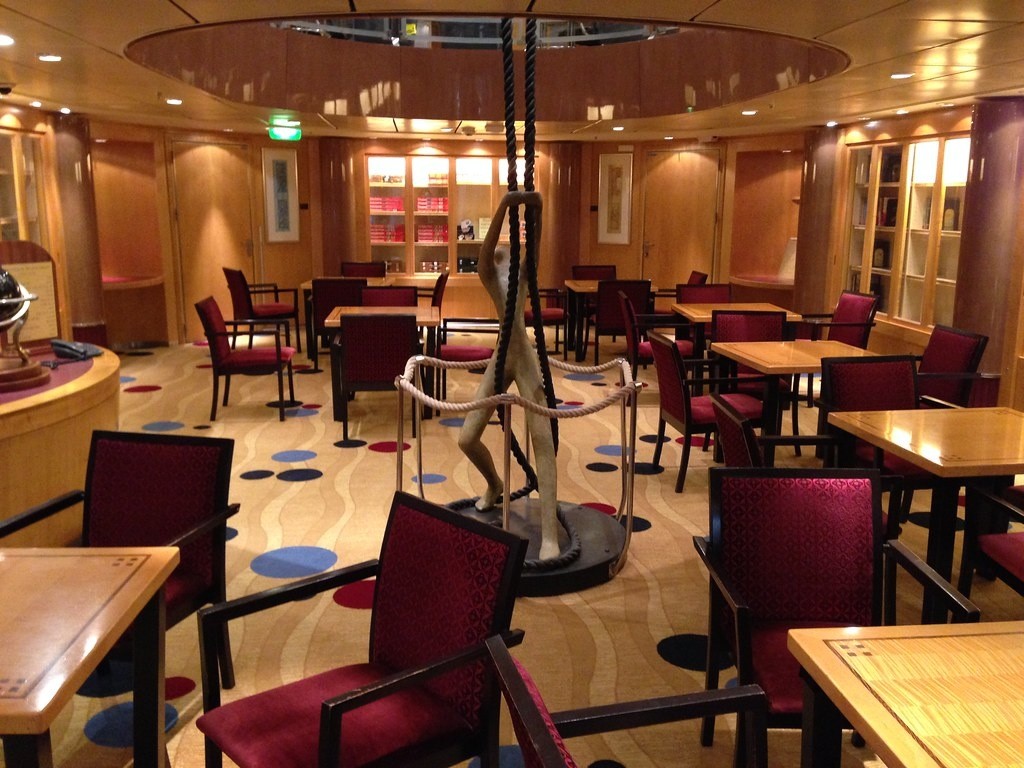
[598,153,633,245]
[262,147,300,244]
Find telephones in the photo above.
[50,338,104,361]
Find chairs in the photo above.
[646,329,763,493]
[222,267,302,353]
[709,392,904,541]
[654,270,708,314]
[571,265,616,343]
[196,491,530,768]
[479,635,769,768]
[676,282,734,350]
[813,355,959,523]
[582,280,655,369]
[703,310,802,456]
[692,466,982,768]
[193,295,296,422]
[957,479,1024,600]
[800,290,882,409]
[0,429,236,690]
[916,323,991,409]
[525,288,571,361]
[617,290,694,406]
[312,262,500,442]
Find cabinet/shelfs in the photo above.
[369,157,533,276]
[846,137,966,327]
[0,128,42,241]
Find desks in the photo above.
[324,306,440,421]
[0,340,121,547]
[711,340,883,468]
[565,280,658,362]
[0,546,181,768]
[671,303,802,396]
[828,406,1024,624]
[786,622,1024,768]
